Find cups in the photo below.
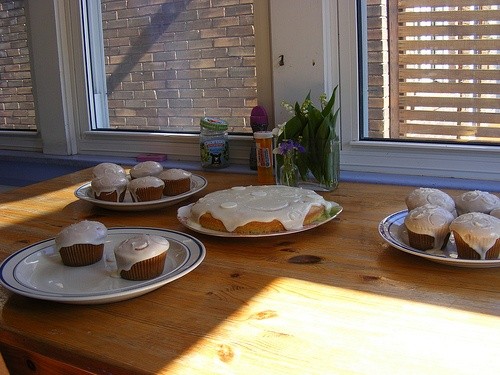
[253,130,273,185]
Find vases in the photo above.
[279,153,300,187]
[274,141,341,191]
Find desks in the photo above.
[0,164,500,375]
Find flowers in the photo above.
[276,86,340,144]
[273,139,306,155]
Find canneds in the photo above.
[199,118,229,168]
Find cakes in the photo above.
[449,211,500,260]
[114,234,170,281]
[129,161,164,180]
[92,162,128,203]
[55,219,107,266]
[159,168,192,196]
[404,204,454,254]
[405,186,455,218]
[455,189,500,217]
[193,184,332,233]
[129,175,165,203]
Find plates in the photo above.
[177,200,343,238]
[74,172,207,210]
[0,226,206,305]
[377,208,500,268]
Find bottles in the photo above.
[250,106,268,171]
